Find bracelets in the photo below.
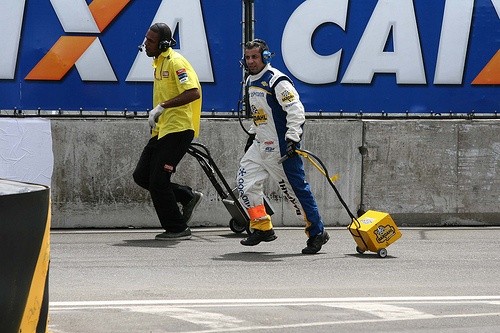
[162,102,166,107]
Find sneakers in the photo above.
[155,227,192,240]
[302,228,330,254]
[181,190,204,225]
[240,228,278,246]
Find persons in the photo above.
[133,24,204,241]
[236,39,330,254]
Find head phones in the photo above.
[238,39,271,68]
[155,23,177,52]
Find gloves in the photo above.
[244,134,255,153]
[286,138,297,159]
[148,104,164,128]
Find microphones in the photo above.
[138,45,144,52]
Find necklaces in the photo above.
[154,58,165,80]
